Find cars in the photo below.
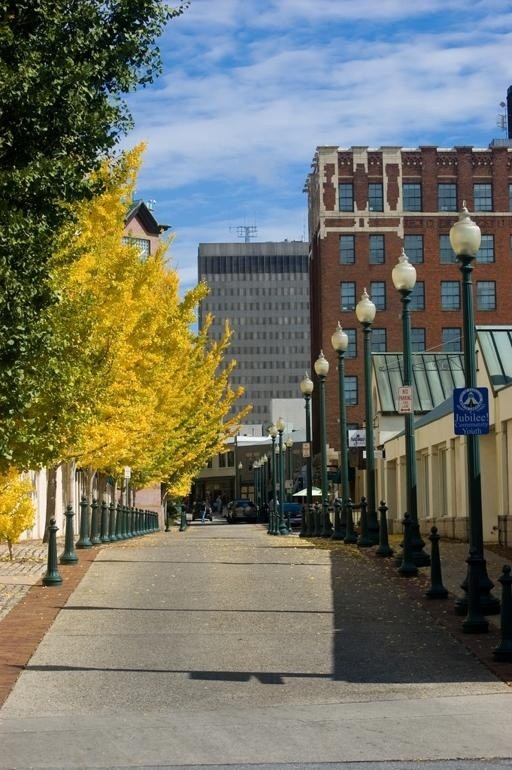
[192,502,213,521]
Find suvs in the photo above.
[224,500,257,524]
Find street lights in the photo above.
[238,461,243,499]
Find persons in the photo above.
[268,495,280,516]
[182,492,222,523]
